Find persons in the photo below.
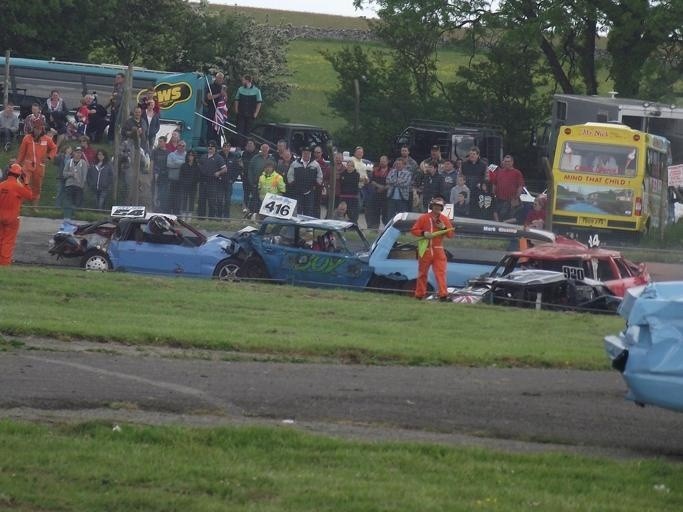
[0,164,33,264]
[410,196,453,301]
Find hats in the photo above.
[299,144,315,152]
[78,135,90,141]
[431,143,440,149]
[9,163,22,175]
[429,197,444,212]
[74,144,84,152]
[33,118,44,129]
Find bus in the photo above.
[544,120,671,244]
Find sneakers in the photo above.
[416,296,425,302]
[439,296,453,303]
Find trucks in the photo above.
[0,56,334,164]
